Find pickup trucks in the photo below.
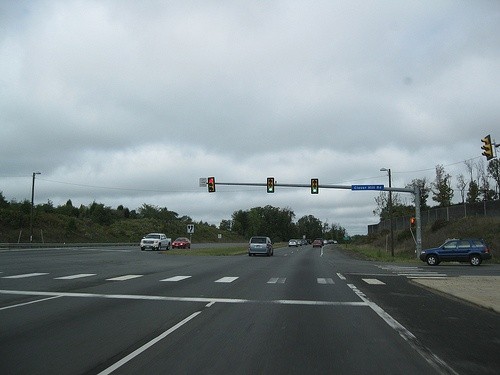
[140,233,172,251]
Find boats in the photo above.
[315,237,338,245]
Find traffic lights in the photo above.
[410,217,416,224]
[267,178,274,193]
[207,177,215,193]
[311,178,319,194]
[481,134,494,161]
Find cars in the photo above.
[313,240,322,248]
[297,238,313,246]
[172,237,191,249]
[289,239,298,247]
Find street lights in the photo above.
[29,172,43,243]
[380,167,394,258]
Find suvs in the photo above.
[420,237,492,266]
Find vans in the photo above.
[248,236,273,257]
